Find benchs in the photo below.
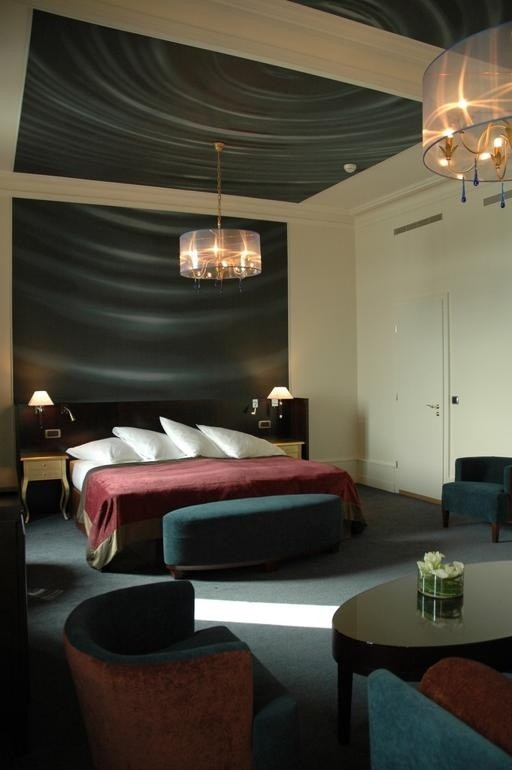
[163,492,342,578]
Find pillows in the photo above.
[67,416,286,464]
[420,655,512,756]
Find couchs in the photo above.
[367,657,512,768]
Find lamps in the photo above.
[422,21,512,208]
[179,143,262,297]
[27,391,55,451]
[267,386,294,436]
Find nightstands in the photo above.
[20,450,70,522]
[264,436,305,461]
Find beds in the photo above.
[47,399,351,569]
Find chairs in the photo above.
[62,580,295,770]
[441,457,512,543]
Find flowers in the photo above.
[418,610,464,629]
[415,551,463,579]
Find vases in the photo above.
[416,569,464,599]
[416,592,464,617]
